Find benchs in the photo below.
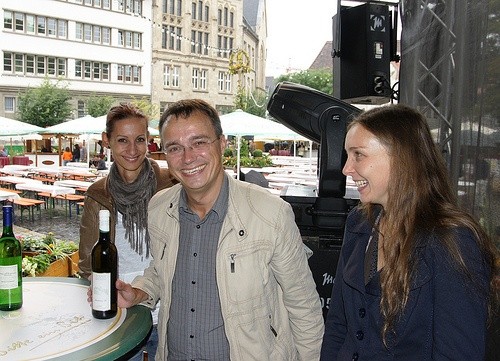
[0,176,89,223]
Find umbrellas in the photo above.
[0,108,312,142]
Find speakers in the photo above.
[333,4,390,105]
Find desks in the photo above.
[0,156,318,224]
[0,276,153,361]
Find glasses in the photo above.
[160,136,220,154]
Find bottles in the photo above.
[0,206,22,311]
[91,209,119,319]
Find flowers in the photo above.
[0,232,77,277]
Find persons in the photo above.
[0,139,305,170]
[320,104,493,361]
[78,102,180,361]
[87,99,326,361]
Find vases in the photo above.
[20,248,80,277]
